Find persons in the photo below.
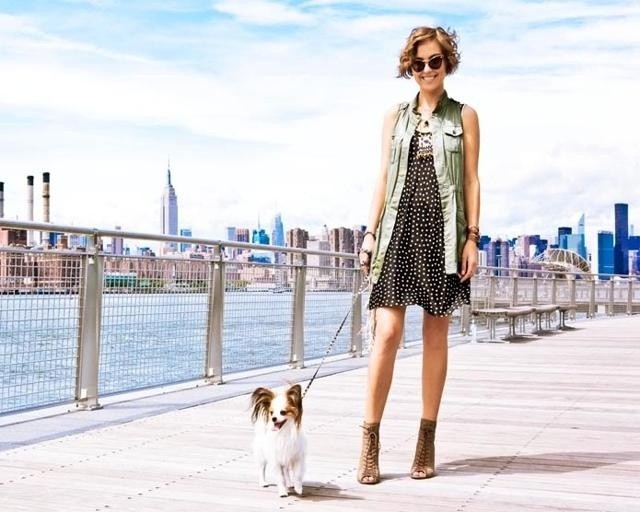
[353,26,481,486]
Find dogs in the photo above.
[245,383,307,497]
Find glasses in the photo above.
[411,55,445,73]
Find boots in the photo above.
[357,421,380,484]
[410,417,436,479]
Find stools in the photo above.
[468,300,579,344]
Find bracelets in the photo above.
[468,224,480,248]
[363,231,376,241]
[357,247,372,259]
